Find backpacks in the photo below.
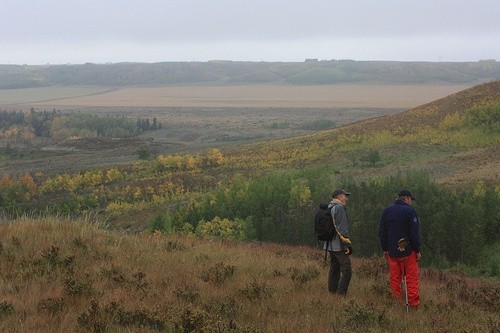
[315,203,339,245]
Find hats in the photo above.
[397,190,415,200]
[332,189,351,198]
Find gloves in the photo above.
[344,244,353,255]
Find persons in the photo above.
[378,190,422,306]
[322,189,353,296]
